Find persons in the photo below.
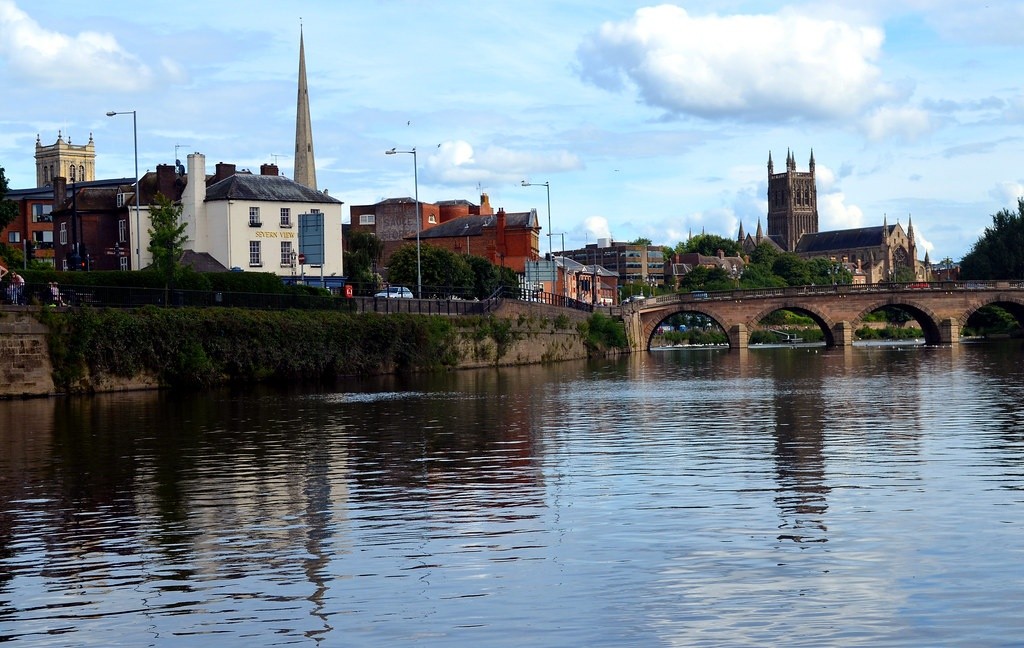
[45,282,68,307]
[6,271,25,306]
[0,265,9,282]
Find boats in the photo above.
[665,341,730,349]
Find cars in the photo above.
[907,282,930,288]
[374,286,414,299]
[690,291,708,298]
[622,294,646,305]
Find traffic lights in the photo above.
[81,260,86,269]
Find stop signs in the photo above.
[299,254,305,263]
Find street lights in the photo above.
[521,180,555,307]
[385,146,423,299]
[548,231,568,308]
[106,108,141,274]
